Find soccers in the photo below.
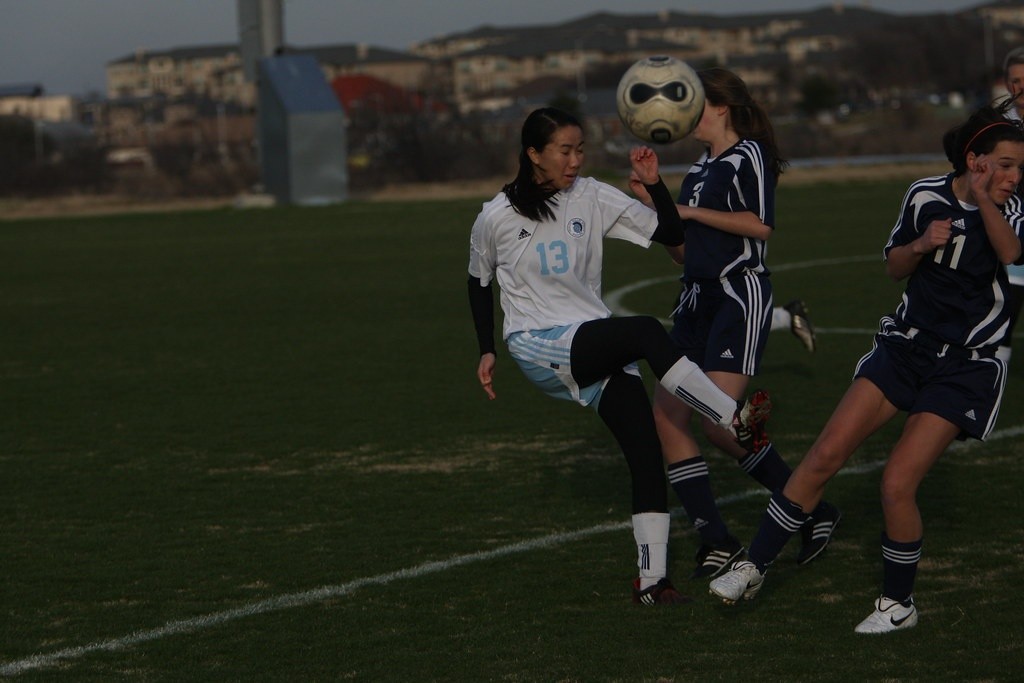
[615,55,705,143]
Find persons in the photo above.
[466,106,772,608]
[706,103,1024,633]
[995,47,1023,362]
[621,67,841,582]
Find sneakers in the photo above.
[732,391,771,453]
[633,577,697,607]
[708,561,767,605]
[795,500,840,566]
[694,535,746,582]
[853,594,917,633]
